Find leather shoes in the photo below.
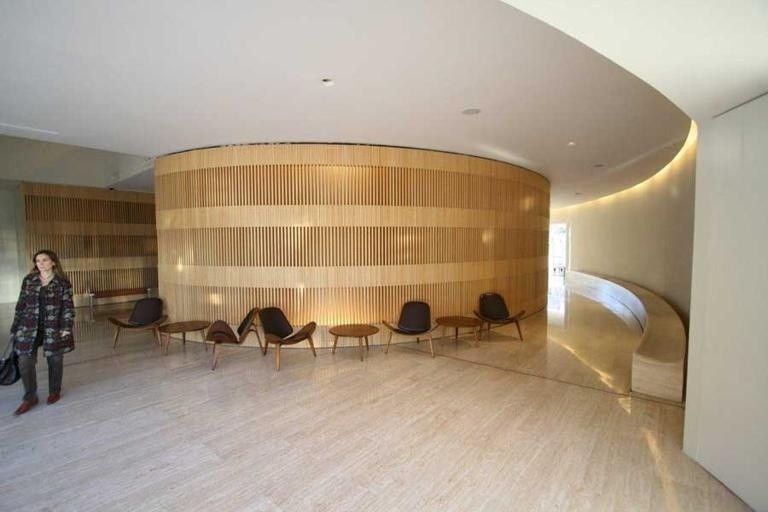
[14,395,40,415]
[46,393,61,403]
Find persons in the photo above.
[8,248,75,416]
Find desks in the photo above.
[329,325,379,361]
[436,316,481,348]
[158,321,210,355]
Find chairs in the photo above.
[474,292,526,341]
[382,301,440,358]
[259,307,317,371]
[107,298,168,349]
[207,308,264,371]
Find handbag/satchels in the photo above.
[0,331,22,386]
